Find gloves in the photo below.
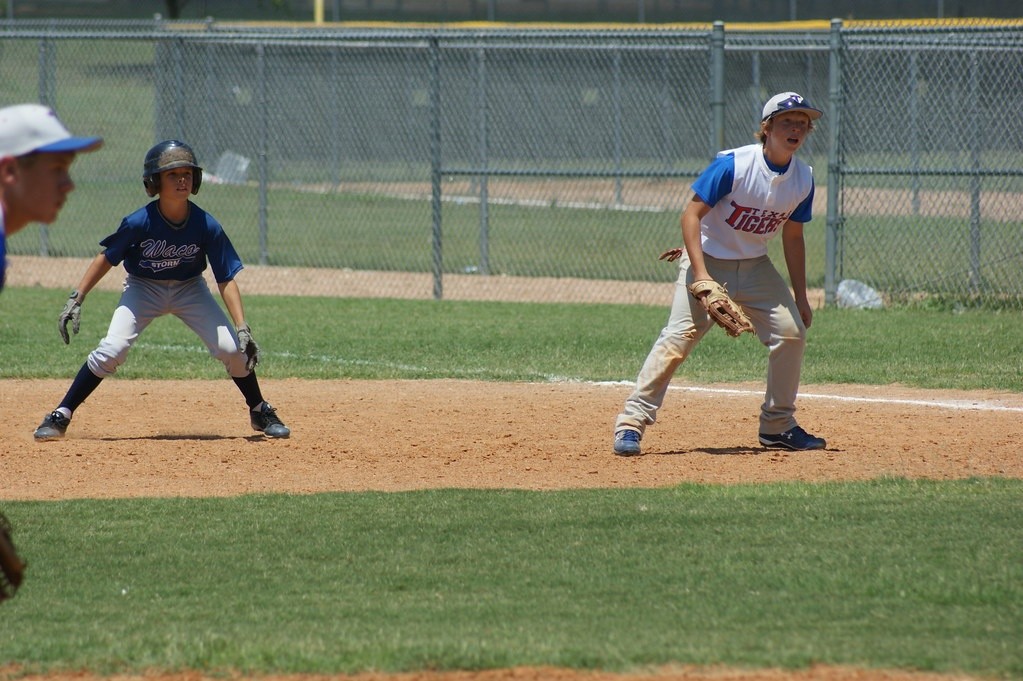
[236,323,260,372]
[58,289,86,345]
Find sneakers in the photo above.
[34,411,70,439]
[759,425,826,451]
[613,429,641,456]
[249,401,290,438]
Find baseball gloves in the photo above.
[688,278,755,338]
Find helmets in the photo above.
[142,141,205,198]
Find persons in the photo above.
[0,105,104,605]
[614,91,826,456]
[34,140,291,443]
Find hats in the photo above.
[0,102,105,161]
[761,91,823,125]
